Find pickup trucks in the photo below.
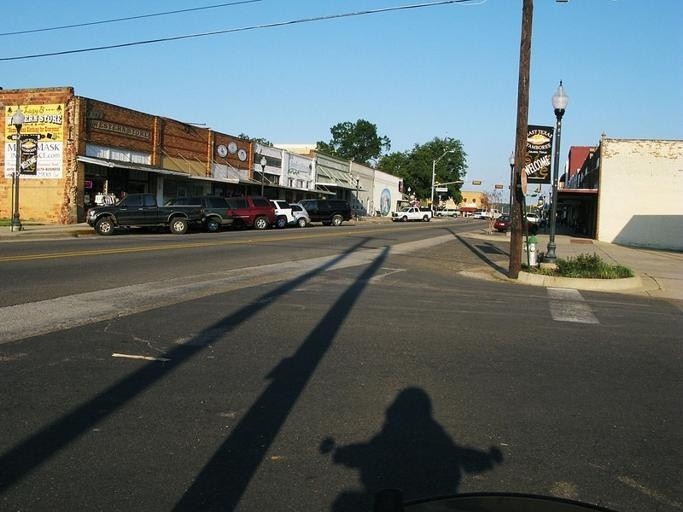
[84,192,204,235]
[390,206,431,222]
[481,208,503,220]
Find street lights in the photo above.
[354,174,360,218]
[407,186,411,203]
[259,155,266,196]
[9,106,24,232]
[542,79,570,262]
[429,150,455,213]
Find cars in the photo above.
[523,213,541,223]
[493,215,537,234]
[288,203,310,227]
[434,207,460,218]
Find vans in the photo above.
[474,209,485,219]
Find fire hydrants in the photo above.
[523,234,540,269]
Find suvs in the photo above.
[269,199,295,229]
[224,196,276,229]
[160,196,234,232]
[297,199,352,226]
